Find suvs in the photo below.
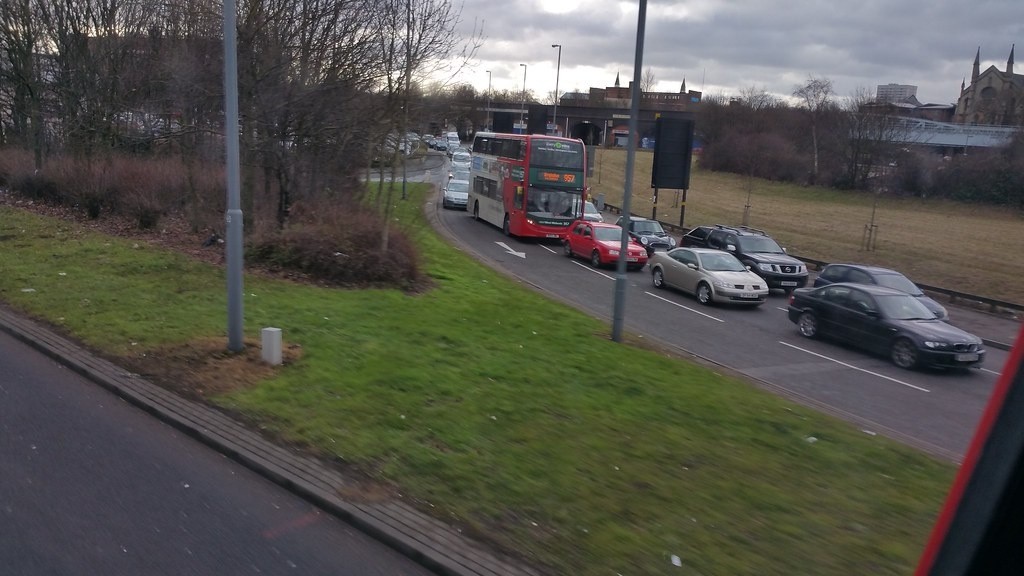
[680,223,809,295]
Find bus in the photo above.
[466,131,591,242]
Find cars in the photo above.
[615,215,676,255]
[815,262,950,322]
[573,199,603,223]
[443,179,470,210]
[563,220,648,271]
[450,151,472,170]
[649,247,769,308]
[449,170,471,180]
[788,283,986,372]
[386,132,467,161]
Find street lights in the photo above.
[486,71,491,129]
[520,63,527,134]
[551,44,563,136]
